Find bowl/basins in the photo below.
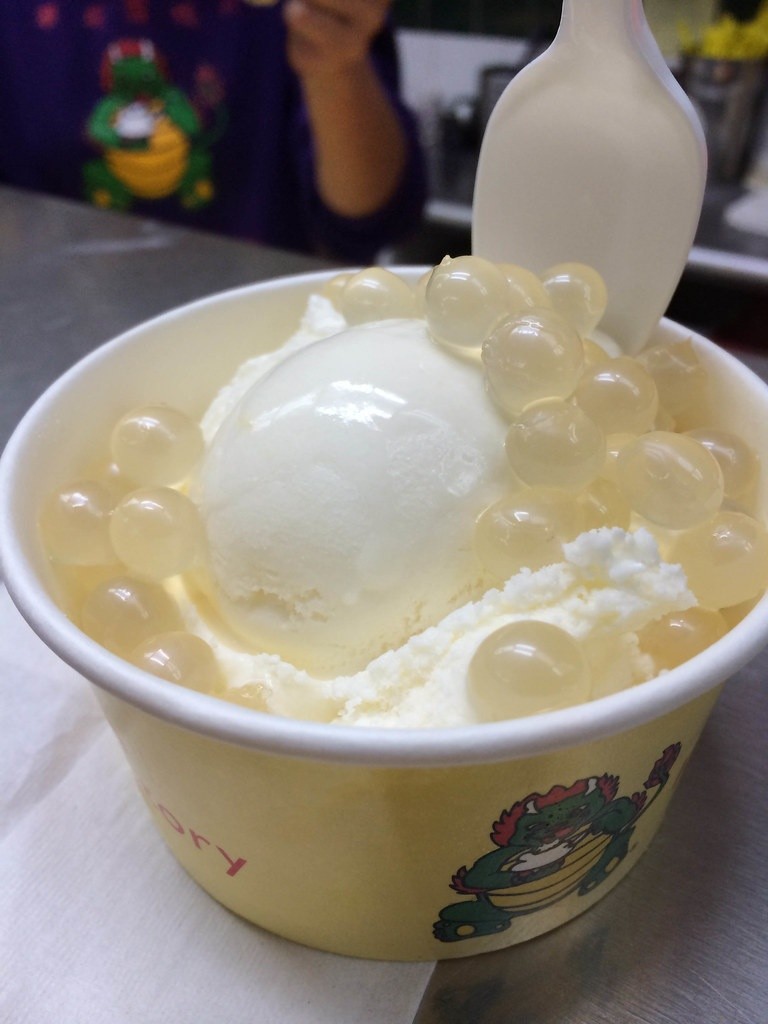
[0,264,768,964]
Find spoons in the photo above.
[470,0,709,353]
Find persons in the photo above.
[2,0,428,262]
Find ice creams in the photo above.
[157,292,704,742]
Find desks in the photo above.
[0,183,768,1024]
